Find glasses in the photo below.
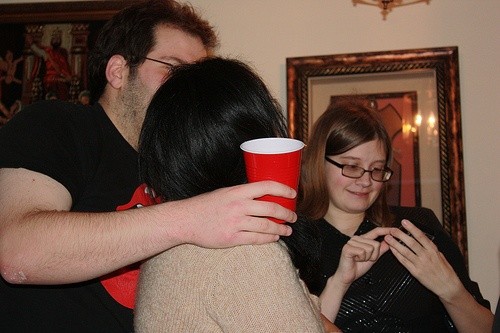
[324,155,393,182]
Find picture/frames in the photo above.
[286,46,469,277]
[0,1,180,127]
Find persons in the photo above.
[1,0,298,333]
[133,55,344,333]
[278,103,495,333]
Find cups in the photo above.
[240,138,305,223]
